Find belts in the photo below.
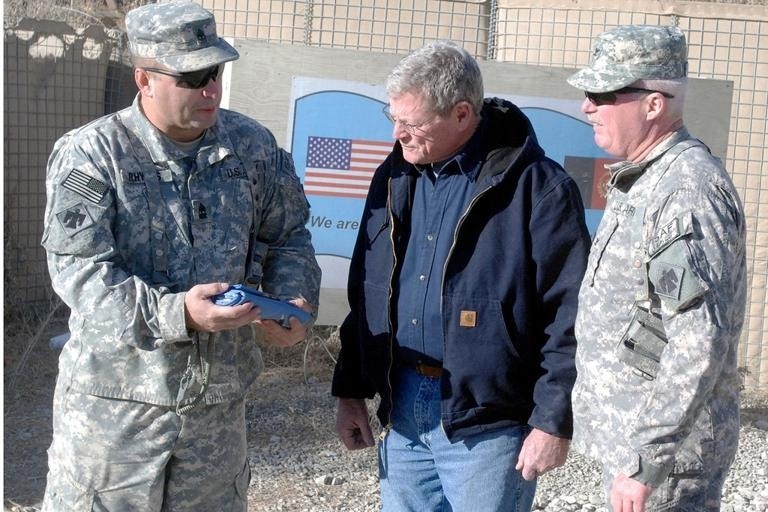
[412,360,443,377]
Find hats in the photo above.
[566,24,688,94]
[125,1,239,74]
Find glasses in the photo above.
[135,62,226,90]
[382,102,448,133]
[583,84,674,105]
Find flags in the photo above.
[565,156,625,210]
[304,136,396,199]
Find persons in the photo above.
[570,27,746,512]
[41,2,321,512]
[331,43,591,512]
